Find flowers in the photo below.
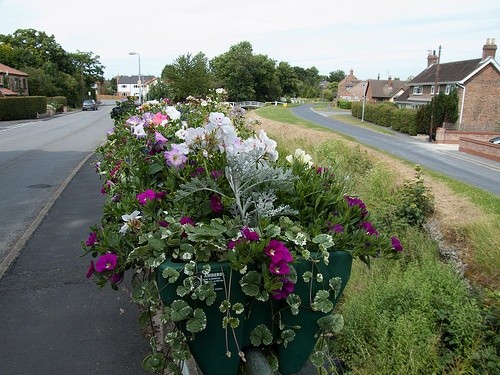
[82,88,403,375]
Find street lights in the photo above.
[138,73,145,104]
[128,52,141,105]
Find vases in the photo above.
[158,246,353,375]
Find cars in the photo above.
[82,99,98,111]
[489,136,500,145]
